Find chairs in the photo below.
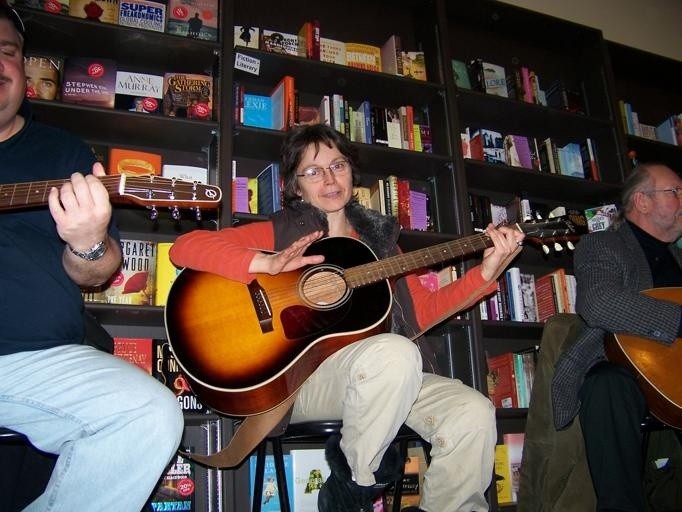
[548,313,672,512]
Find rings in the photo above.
[517,240,523,246]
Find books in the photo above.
[248,433,526,512]
[79,239,184,307]
[13,2,219,188]
[413,263,577,407]
[453,59,621,234]
[232,20,440,233]
[619,101,682,145]
[106,336,214,511]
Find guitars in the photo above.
[605,286,681,429]
[165,209,586,418]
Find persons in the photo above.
[549,162,682,512]
[2,1,185,511]
[168,124,525,512]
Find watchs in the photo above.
[66,240,107,260]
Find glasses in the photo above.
[295,156,351,182]
[653,184,681,200]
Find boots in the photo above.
[318,436,404,512]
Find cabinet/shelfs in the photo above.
[603,38,682,248]
[0,0,602,512]
